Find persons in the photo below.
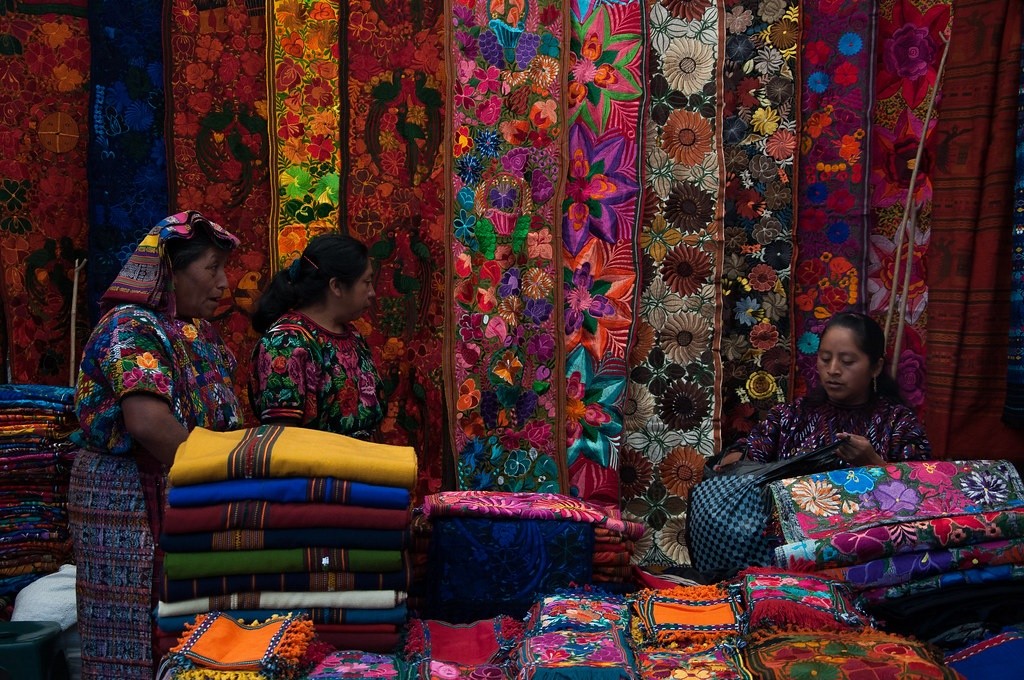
[246,231,387,443]
[68,208,244,680]
[711,311,930,473]
